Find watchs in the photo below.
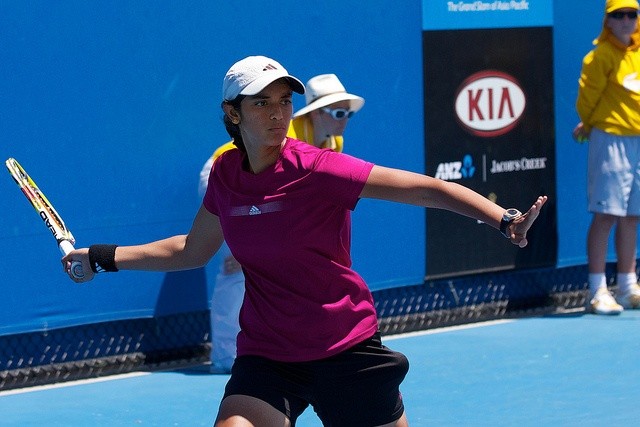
[498,208,522,239]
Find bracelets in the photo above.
[88,244,119,274]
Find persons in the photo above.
[61,54,549,427]
[573,0,640,314]
[199,73,365,375]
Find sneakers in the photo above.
[210,357,235,374]
[615,285,640,308]
[585,288,624,315]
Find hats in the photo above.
[604,0,640,13]
[223,55,306,102]
[294,73,364,118]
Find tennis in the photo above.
[576,131,586,144]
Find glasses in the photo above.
[609,12,637,19]
[322,107,354,121]
